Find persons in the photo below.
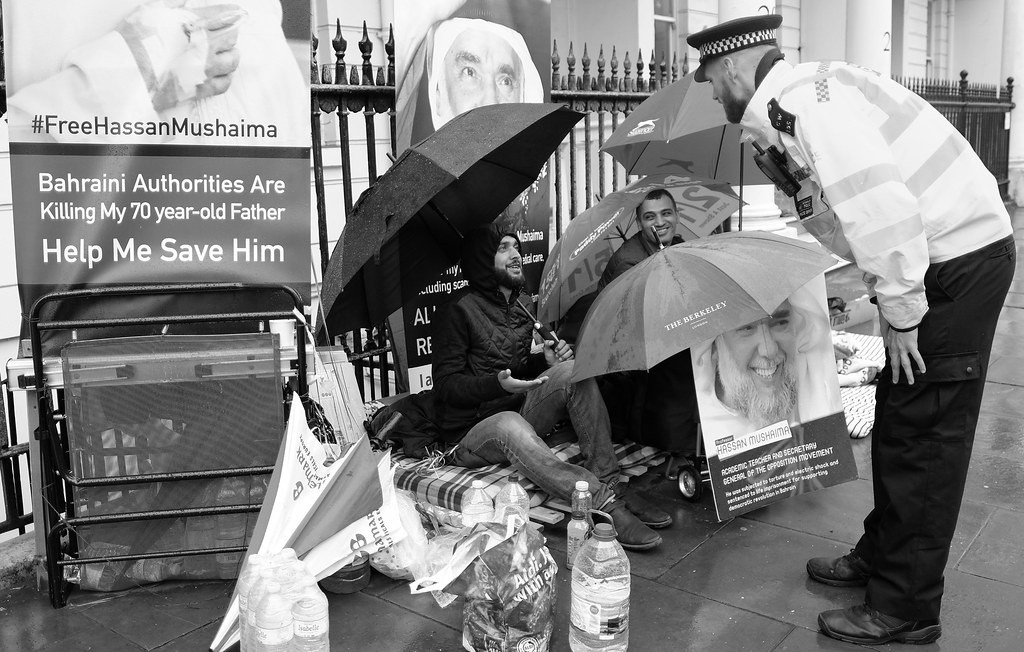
[690,285,830,432]
[432,0,545,119]
[685,13,1017,643]
[5,0,284,144]
[431,188,885,554]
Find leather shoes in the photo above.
[818,602,942,645]
[807,549,872,586]
[616,483,673,528]
[595,499,662,550]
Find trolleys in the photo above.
[666,419,711,503]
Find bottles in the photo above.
[566,511,589,571]
[494,475,529,524]
[460,479,493,529]
[238,546,330,652]
[571,480,593,531]
[569,508,630,652]
[462,504,556,652]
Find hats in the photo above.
[686,14,783,83]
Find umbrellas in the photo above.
[572,223,839,382]
[539,172,748,325]
[314,103,587,354]
[208,391,408,652]
[598,66,776,231]
